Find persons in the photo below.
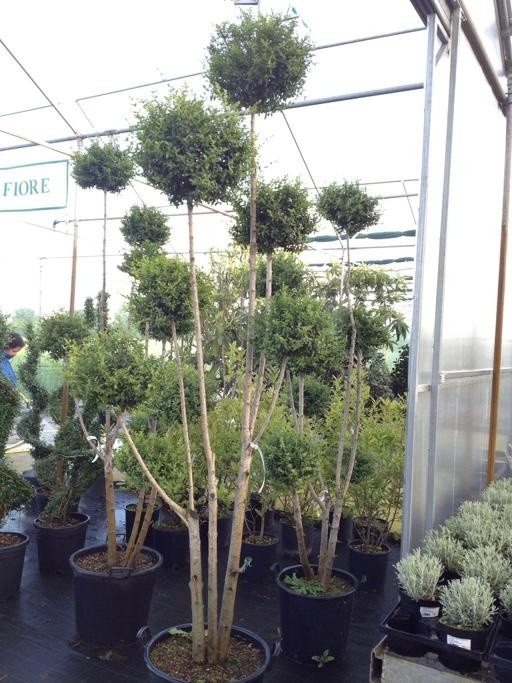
[0,331,26,387]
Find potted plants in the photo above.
[2,12,510,683]
